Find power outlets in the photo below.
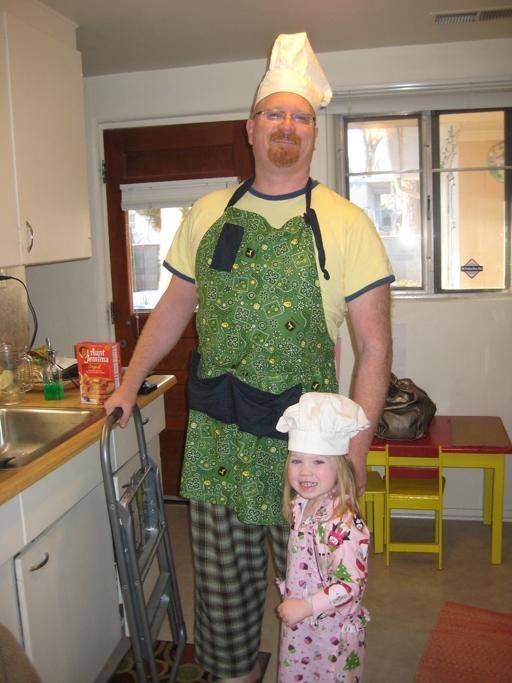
[0,271,7,288]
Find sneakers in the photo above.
[198,660,261,683]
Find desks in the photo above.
[366,416,512,565]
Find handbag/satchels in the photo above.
[374,373,436,440]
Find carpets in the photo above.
[109,641,272,683]
[412,600,512,682]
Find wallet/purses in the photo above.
[138,383,157,394]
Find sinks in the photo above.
[0,404,109,467]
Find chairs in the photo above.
[358,470,385,555]
[385,444,445,570]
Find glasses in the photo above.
[253,109,315,125]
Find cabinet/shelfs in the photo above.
[112,392,167,640]
[0,1,93,268]
[0,433,127,683]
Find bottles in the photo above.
[1,343,16,369]
[13,352,32,393]
[43,349,64,400]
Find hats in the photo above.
[254,32,333,113]
[276,392,371,456]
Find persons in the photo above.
[275,449,370,683]
[102,92,396,683]
